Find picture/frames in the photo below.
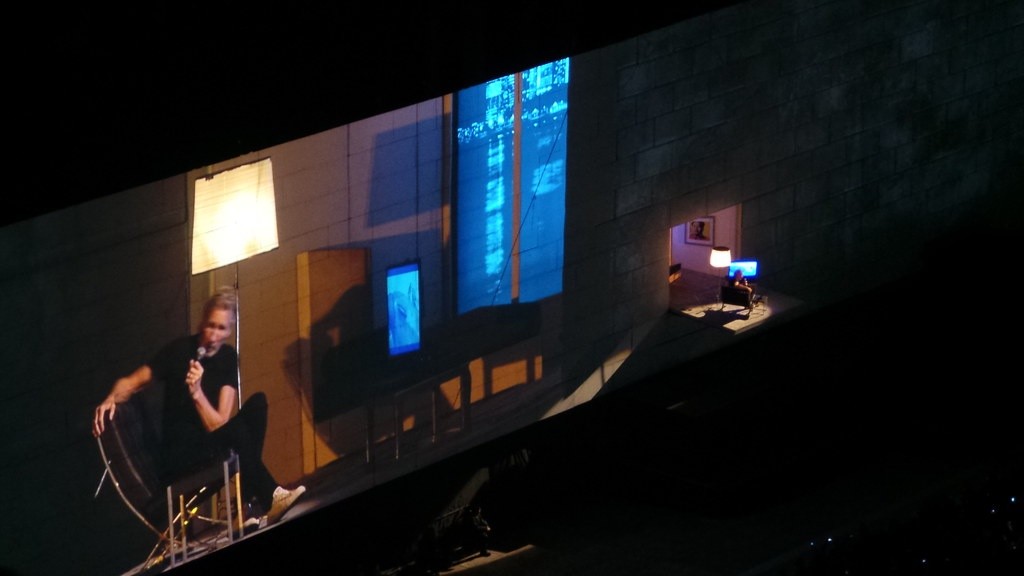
[685,216,716,247]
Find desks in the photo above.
[364,364,475,467]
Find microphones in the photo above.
[195,347,206,362]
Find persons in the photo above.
[93,286,306,541]
[689,221,710,240]
[729,270,756,306]
[472,507,492,556]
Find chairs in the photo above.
[96,399,243,574]
[721,286,754,318]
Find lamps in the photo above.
[710,246,733,309]
[192,159,282,527]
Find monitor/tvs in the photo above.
[386,258,421,357]
[728,261,758,279]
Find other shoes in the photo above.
[266,485,305,526]
[227,513,260,538]
